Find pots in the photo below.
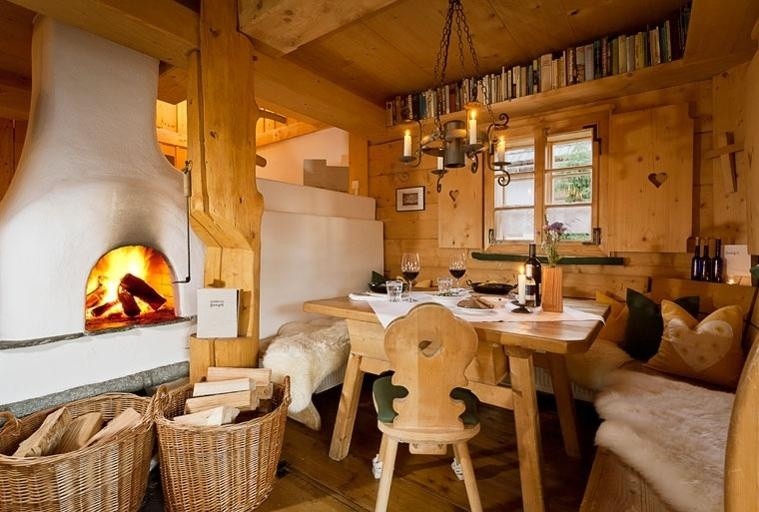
[467,279,519,295]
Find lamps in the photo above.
[398,0,513,193]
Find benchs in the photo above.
[260,317,350,430]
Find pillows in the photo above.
[640,298,744,392]
[616,288,700,364]
[592,290,628,343]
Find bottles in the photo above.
[711,238,723,283]
[700,237,712,281]
[523,242,542,308]
[690,236,702,281]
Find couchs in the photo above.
[500,271,758,512]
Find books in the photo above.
[383,5,691,129]
[719,243,752,278]
[196,287,243,339]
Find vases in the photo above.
[541,264,563,312]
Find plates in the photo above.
[368,284,405,293]
[456,305,495,312]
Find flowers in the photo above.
[534,221,569,267]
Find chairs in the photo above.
[371,302,484,511]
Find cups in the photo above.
[436,276,453,295]
[385,279,403,303]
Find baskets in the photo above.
[154,376,290,512]
[0,391,153,512]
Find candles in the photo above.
[518,275,526,304]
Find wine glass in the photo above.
[447,251,467,294]
[400,252,421,304]
